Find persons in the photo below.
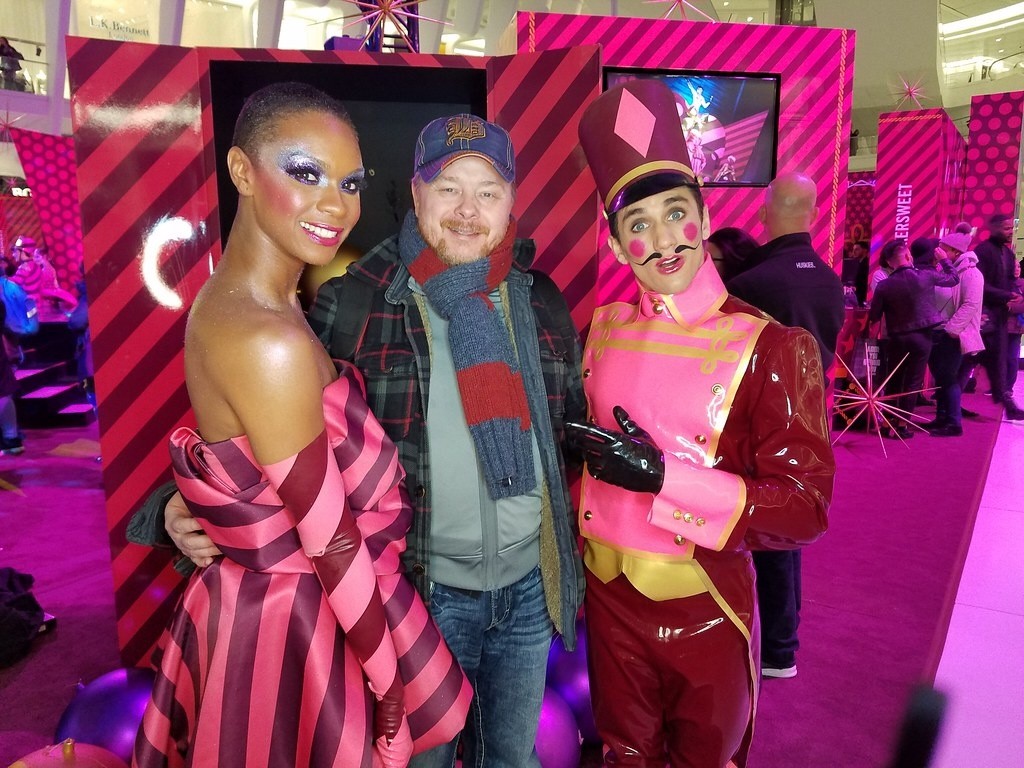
[869,242,960,439]
[725,172,844,678]
[561,80,835,768]
[852,214,1024,420]
[704,227,762,281]
[920,233,986,437]
[0,234,98,455]
[126,112,589,768]
[0,36,25,91]
[133,82,473,768]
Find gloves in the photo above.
[561,405,666,497]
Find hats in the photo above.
[577,78,705,221]
[10,233,38,257]
[938,232,973,253]
[908,236,942,259]
[413,113,516,185]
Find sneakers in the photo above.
[983,388,993,396]
[960,407,979,418]
[931,425,963,437]
[761,653,798,679]
[919,418,947,429]
[879,426,892,438]
[892,426,915,440]
[999,390,1024,420]
